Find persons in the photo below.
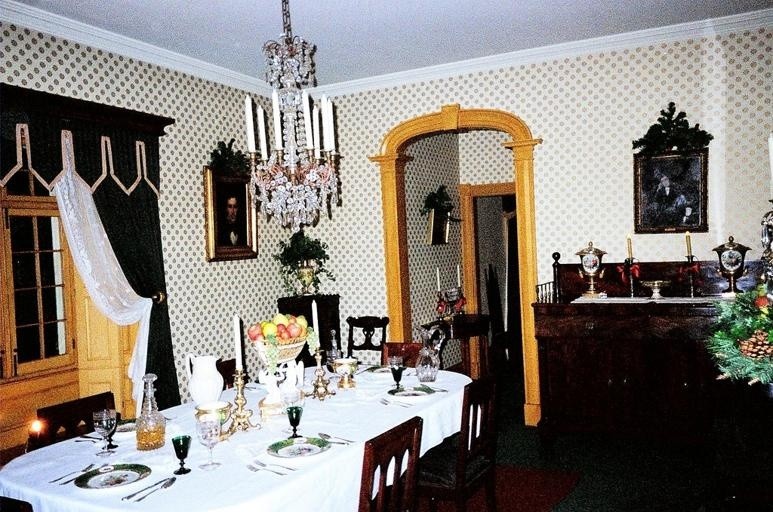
[219,192,244,247]
[655,174,691,227]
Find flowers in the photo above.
[700,279,773,387]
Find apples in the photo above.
[289,315,297,323]
[248,323,262,340]
[276,323,302,340]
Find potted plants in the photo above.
[273,229,338,296]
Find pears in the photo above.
[263,322,278,338]
[297,315,308,327]
[261,321,267,327]
[273,313,288,327]
[300,326,307,337]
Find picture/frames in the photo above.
[201,163,259,262]
[427,206,450,245]
[630,151,709,234]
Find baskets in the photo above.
[254,333,307,366]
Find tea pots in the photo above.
[413,323,445,382]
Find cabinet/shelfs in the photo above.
[531,290,746,441]
[276,294,342,368]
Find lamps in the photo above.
[241,0,343,235]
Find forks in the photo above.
[245,458,296,479]
[51,461,109,487]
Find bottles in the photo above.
[136,373,165,452]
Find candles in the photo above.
[627,236,632,260]
[435,265,440,292]
[233,313,246,374]
[683,230,693,256]
[456,263,461,288]
[311,300,321,350]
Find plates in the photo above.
[364,366,393,374]
[388,387,430,399]
[267,437,330,460]
[72,462,154,495]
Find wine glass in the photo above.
[93,410,121,457]
[195,415,224,473]
[386,366,405,395]
[169,436,191,475]
[283,406,304,440]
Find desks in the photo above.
[421,314,492,383]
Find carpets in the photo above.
[371,460,585,512]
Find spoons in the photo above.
[317,431,356,445]
[135,477,179,502]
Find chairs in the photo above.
[344,313,389,365]
[355,412,425,512]
[189,354,248,394]
[422,376,496,512]
[33,389,119,455]
[379,340,423,369]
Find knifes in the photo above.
[121,477,169,502]
